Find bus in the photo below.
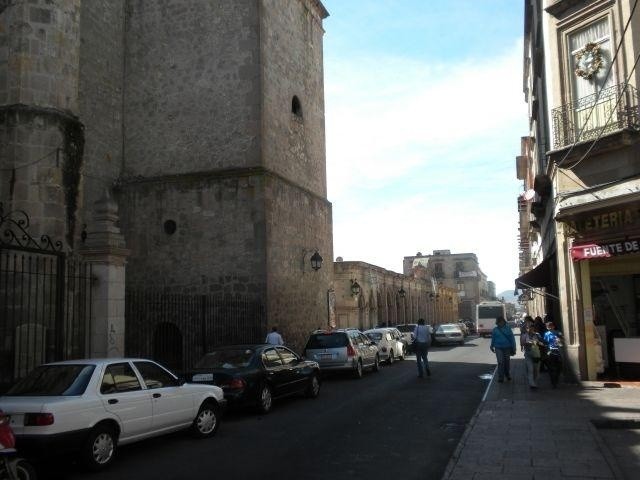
[475,300,507,338]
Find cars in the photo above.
[0,357,228,469]
[363,319,475,366]
[507,312,521,328]
[186,344,322,414]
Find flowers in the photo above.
[574,41,602,80]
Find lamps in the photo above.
[448,296,453,303]
[302,246,323,277]
[426,291,439,302]
[397,288,406,298]
[350,278,361,298]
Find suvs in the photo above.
[300,327,380,380]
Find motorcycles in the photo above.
[0,408,39,480]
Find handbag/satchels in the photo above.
[412,341,417,353]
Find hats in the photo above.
[496,317,506,324]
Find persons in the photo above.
[412,318,433,379]
[520,316,563,389]
[265,326,285,346]
[458,318,475,336]
[490,317,516,383]
